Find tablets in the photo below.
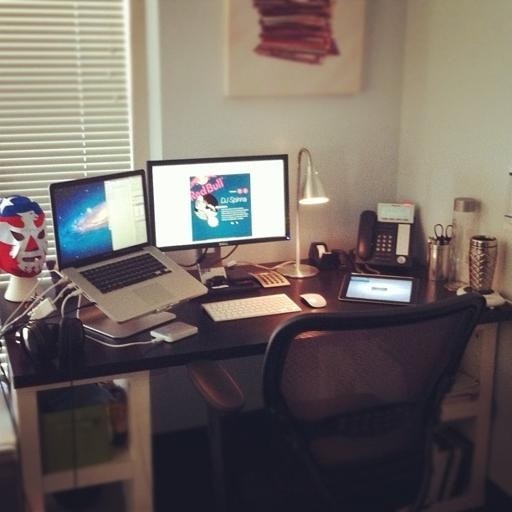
[338,272,417,307]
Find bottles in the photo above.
[449,197,477,288]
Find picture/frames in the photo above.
[224,0,368,96]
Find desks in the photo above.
[0,256,510,512]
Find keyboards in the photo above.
[201,293,303,322]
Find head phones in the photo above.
[16,317,84,362]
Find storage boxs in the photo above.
[37,386,118,471]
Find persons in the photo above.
[0,195,50,303]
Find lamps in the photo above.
[279,145,330,279]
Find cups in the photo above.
[425,242,452,283]
[468,236,498,291]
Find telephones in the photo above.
[355,202,419,273]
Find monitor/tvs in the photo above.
[146,154,289,298]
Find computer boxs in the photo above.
[37,386,125,512]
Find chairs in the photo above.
[187,292,486,512]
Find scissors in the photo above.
[434,224,454,245]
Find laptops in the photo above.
[48,169,209,324]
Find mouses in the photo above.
[300,293,327,308]
